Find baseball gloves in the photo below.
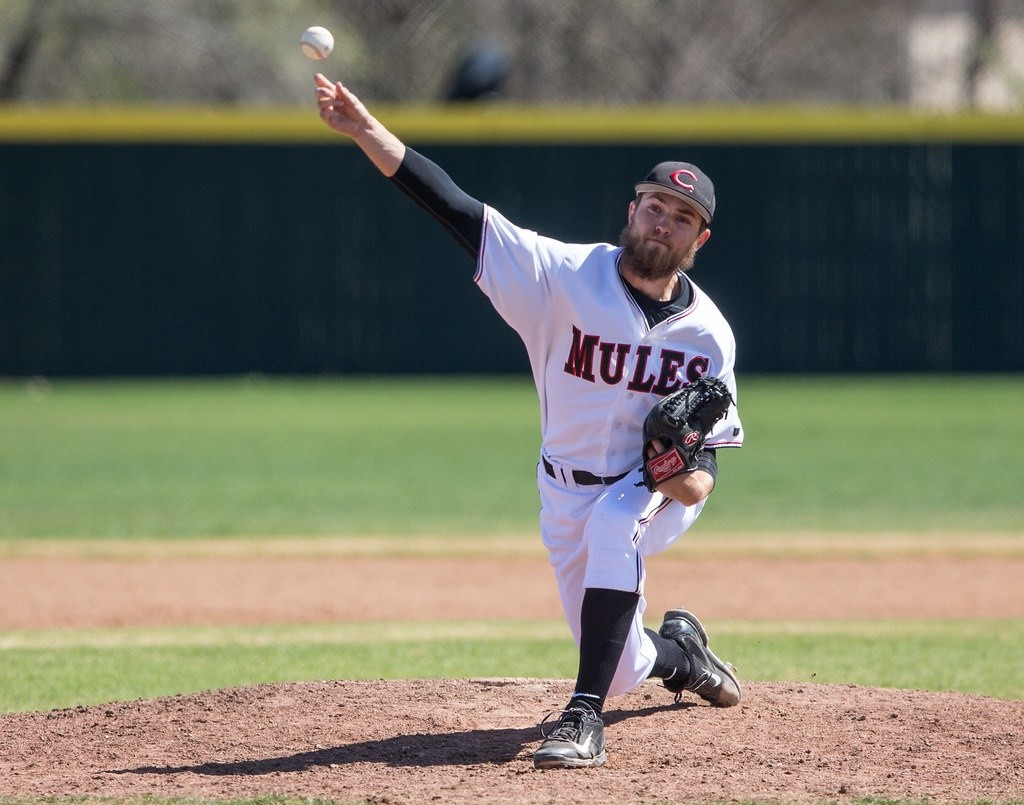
[641,376,732,493]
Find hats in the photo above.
[635,160,716,227]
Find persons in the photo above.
[311,71,744,767]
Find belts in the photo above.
[542,455,629,486]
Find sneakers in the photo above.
[659,609,742,706]
[533,701,607,769]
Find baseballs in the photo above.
[301,25,334,61]
